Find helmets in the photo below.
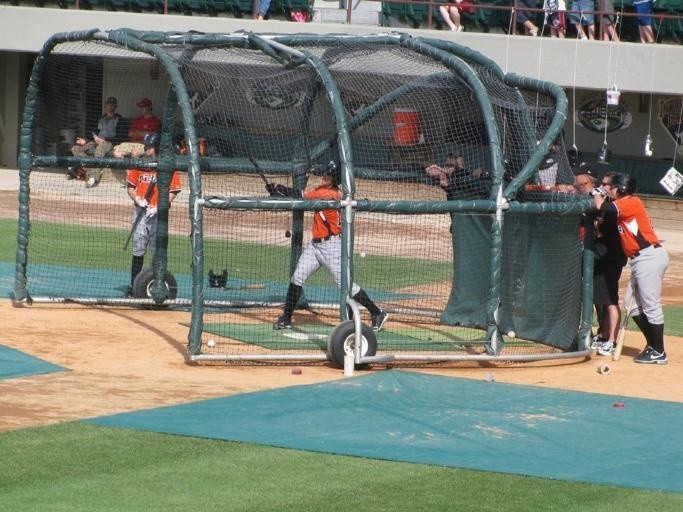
[326,159,342,186]
[608,172,638,198]
[209,271,228,287]
[144,132,161,145]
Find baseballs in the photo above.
[208,339,215,346]
[508,330,516,338]
[360,251,367,258]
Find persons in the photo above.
[252,1,272,21]
[71,97,127,188]
[267,160,390,335]
[551,170,670,366]
[512,1,655,43]
[123,131,181,298]
[113,98,160,157]
[438,1,473,32]
[424,151,466,190]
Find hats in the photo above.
[106,97,116,103]
[577,166,596,176]
[138,98,152,107]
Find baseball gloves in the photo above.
[208,269,228,287]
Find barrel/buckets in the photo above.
[59,129,74,144]
[392,106,422,144]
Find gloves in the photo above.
[146,207,157,218]
[589,188,605,196]
[134,196,148,207]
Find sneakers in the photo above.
[634,345,668,364]
[592,333,616,356]
[371,309,388,331]
[273,315,292,330]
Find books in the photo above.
[660,166,683,196]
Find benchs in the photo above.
[77,147,141,187]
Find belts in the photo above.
[313,238,328,241]
[634,244,659,256]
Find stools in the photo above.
[0,0,682,43]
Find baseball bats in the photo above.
[247,151,270,184]
[613,281,638,361]
[122,179,155,250]
[221,284,266,291]
